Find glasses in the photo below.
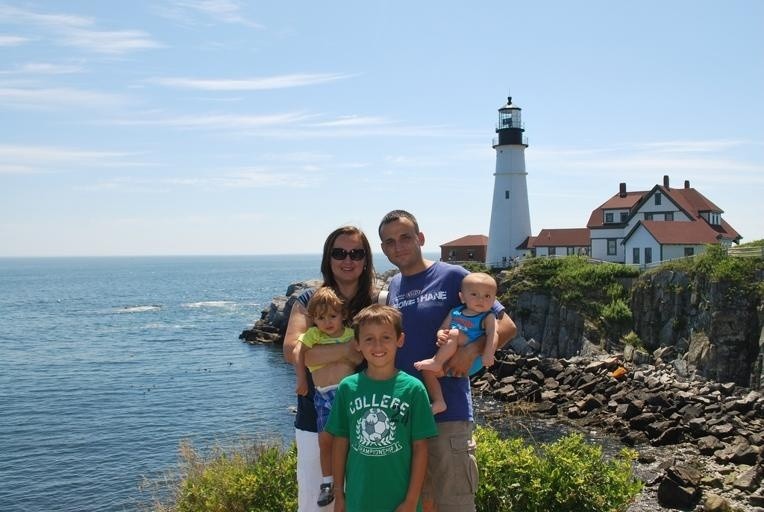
[331,248,367,260]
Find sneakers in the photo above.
[318,483,334,506]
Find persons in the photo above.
[377,209,518,511]
[413,269,499,416]
[291,284,365,506]
[324,302,441,512]
[282,225,388,512]
